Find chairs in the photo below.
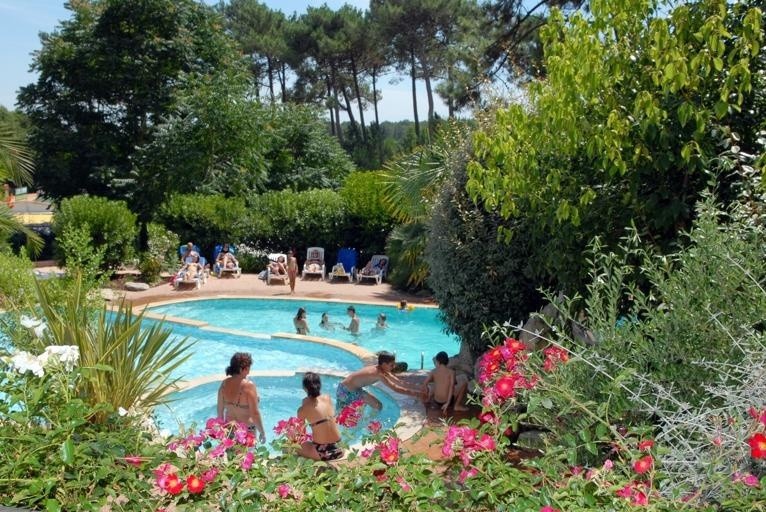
[172,243,390,288]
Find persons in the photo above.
[336,350,427,422]
[176,241,388,296]
[292,371,346,461]
[217,352,268,450]
[375,312,389,332]
[319,312,340,332]
[341,304,359,335]
[291,303,312,336]
[418,348,469,412]
[398,299,408,311]
